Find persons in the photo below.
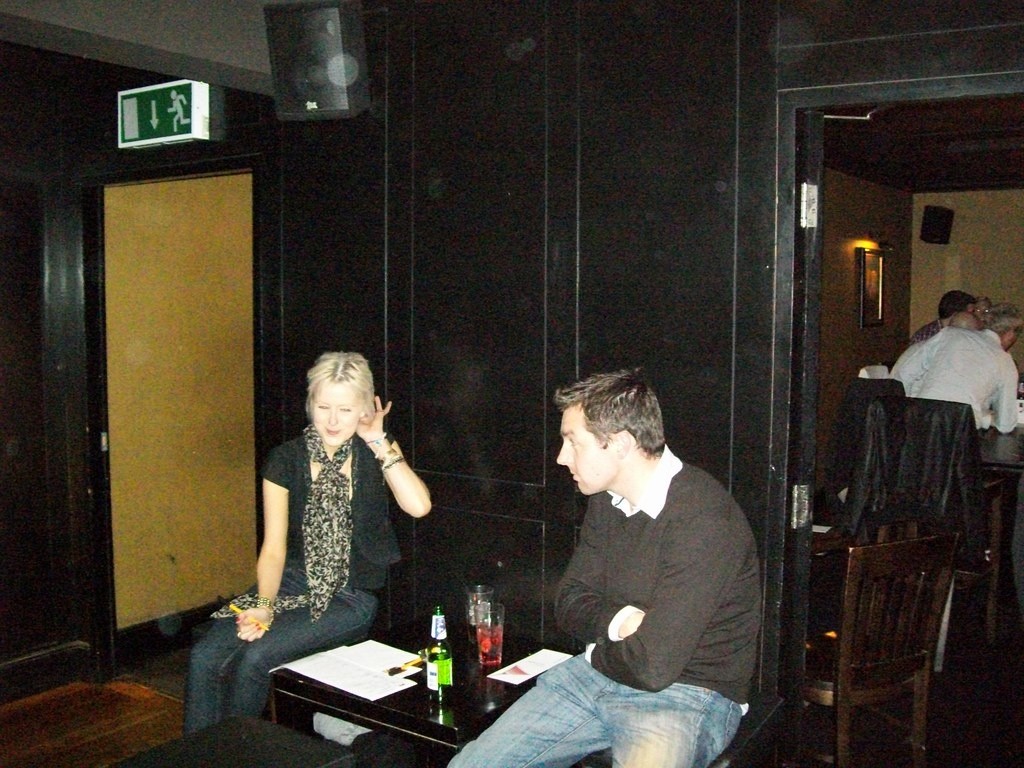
[451,370,762,768]
[892,289,1019,434]
[181,350,431,733]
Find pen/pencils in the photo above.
[215,594,271,633]
[388,658,425,676]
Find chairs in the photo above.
[799,532,957,766]
[812,364,1024,681]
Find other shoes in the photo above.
[954,557,994,576]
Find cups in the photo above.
[475,603,505,667]
[466,584,495,643]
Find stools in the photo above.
[102,714,357,768]
[571,687,784,768]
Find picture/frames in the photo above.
[857,247,886,331]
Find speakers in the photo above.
[264,0,372,120]
[919,205,955,244]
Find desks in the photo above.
[812,525,851,555]
[266,618,585,768]
[976,422,1024,473]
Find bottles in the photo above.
[425,604,454,715]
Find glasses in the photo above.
[975,306,990,315]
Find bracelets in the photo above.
[381,450,407,475]
[256,597,274,628]
[365,439,386,445]
